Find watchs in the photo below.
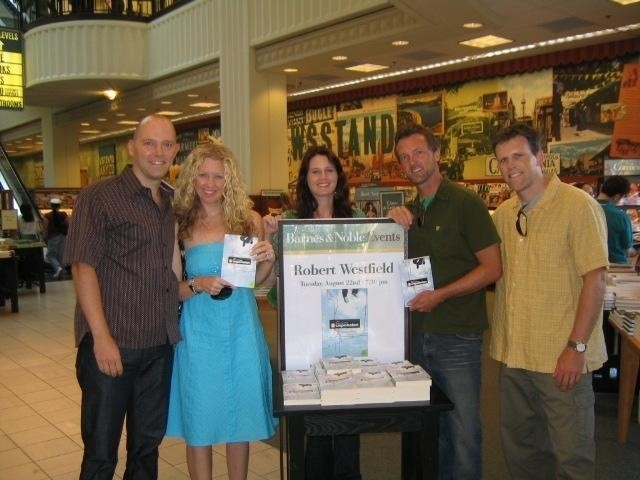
[566,339,589,354]
[189,276,199,297]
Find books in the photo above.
[603,263,640,341]
[221,234,260,289]
[280,357,432,407]
[399,254,434,309]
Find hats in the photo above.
[50,198,62,204]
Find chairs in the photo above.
[0,251,18,312]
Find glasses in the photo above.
[516,201,528,238]
[211,285,233,300]
[418,197,426,228]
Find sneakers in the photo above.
[53,267,63,278]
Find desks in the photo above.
[7,239,47,293]
[608,309,640,442]
[270,355,454,480]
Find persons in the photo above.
[387,124,503,479]
[64,115,181,480]
[44,197,70,277]
[593,173,632,268]
[491,121,609,479]
[165,140,279,480]
[261,145,366,313]
[18,202,42,288]
